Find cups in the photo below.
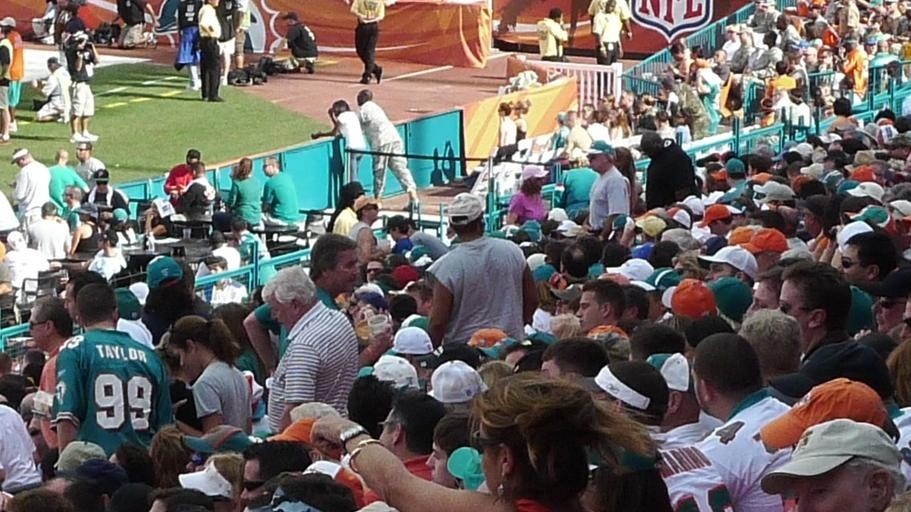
[368,313,389,336]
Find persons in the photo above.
[1,0,910,510]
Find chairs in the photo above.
[472,121,690,196]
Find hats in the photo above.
[6,123,911,511]
[48,57,65,67]
[736,25,754,35]
[1,17,17,28]
[804,47,817,55]
[282,11,299,22]
[866,33,880,45]
[808,2,823,9]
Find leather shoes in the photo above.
[375,66,383,85]
[202,96,224,102]
[359,73,373,85]
